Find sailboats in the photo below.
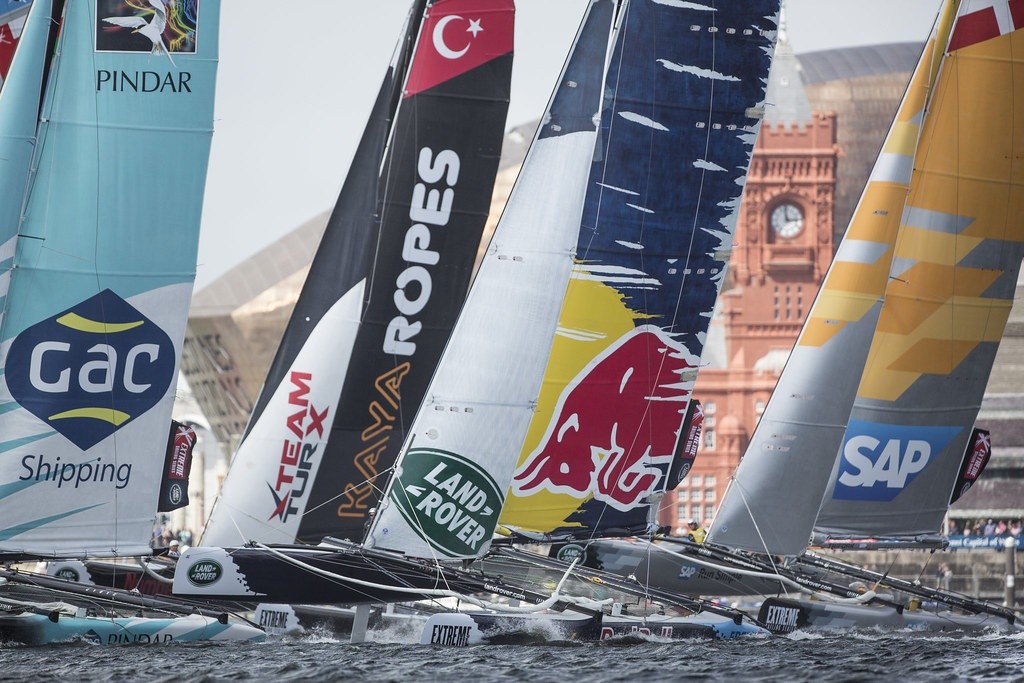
[0,0,268,646]
[171,0,775,649]
[546,0,1024,645]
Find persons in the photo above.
[951,518,1024,536]
[686,519,707,543]
[151,526,193,548]
[168,540,181,557]
[936,562,952,590]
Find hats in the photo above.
[170,540,179,547]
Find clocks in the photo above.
[770,204,803,239]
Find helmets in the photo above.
[687,519,698,529]
